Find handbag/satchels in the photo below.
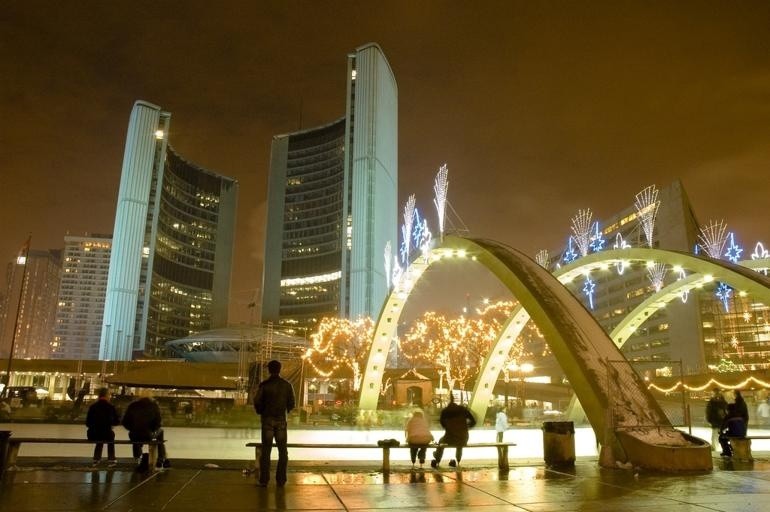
[378,438,400,447]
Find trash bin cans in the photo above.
[541,421,576,468]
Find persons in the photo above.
[85,388,121,468]
[254,359,296,488]
[432,390,476,466]
[733,388,749,436]
[757,399,770,427]
[706,386,729,452]
[121,387,173,471]
[404,408,434,471]
[495,406,509,443]
[717,403,746,456]
[183,402,193,422]
[169,397,178,418]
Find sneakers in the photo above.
[720,451,732,456]
[456,455,461,462]
[256,482,267,487]
[433,452,441,462]
[92,460,118,467]
[729,445,733,451]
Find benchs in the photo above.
[722,435,770,468]
[245,444,516,481]
[7,438,160,474]
